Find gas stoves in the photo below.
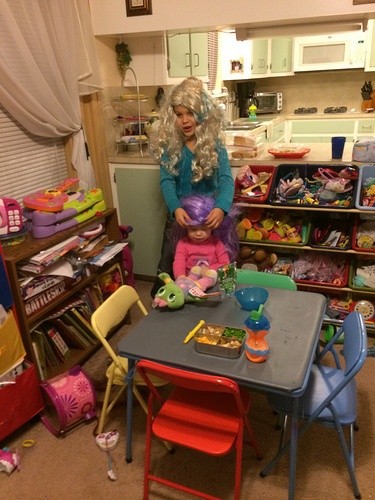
[287,105,355,119]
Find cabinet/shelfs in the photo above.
[122,21,374,335]
[0,204,131,396]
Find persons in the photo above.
[151,79,238,300]
[174,195,229,297]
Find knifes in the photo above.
[361,81,372,100]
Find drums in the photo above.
[38,363,98,436]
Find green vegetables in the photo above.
[222,328,246,340]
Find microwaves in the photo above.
[292,30,368,73]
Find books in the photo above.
[31,307,97,379]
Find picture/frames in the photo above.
[126,0,152,16]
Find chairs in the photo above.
[258,315,363,500]
[135,357,262,500]
[237,269,296,290]
[90,285,175,455]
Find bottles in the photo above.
[242,305,271,363]
[248,104,257,119]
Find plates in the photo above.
[269,148,310,157]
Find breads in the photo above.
[231,134,257,159]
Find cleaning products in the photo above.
[248,103,257,121]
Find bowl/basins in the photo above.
[193,323,247,359]
[235,287,269,310]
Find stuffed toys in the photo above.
[273,254,321,280]
[152,265,221,308]
[239,245,278,270]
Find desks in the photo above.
[117,280,326,500]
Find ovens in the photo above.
[244,91,282,114]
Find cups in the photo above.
[331,136,346,159]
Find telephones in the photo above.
[0,194,23,240]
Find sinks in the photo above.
[225,121,258,127]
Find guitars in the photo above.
[23,174,80,213]
